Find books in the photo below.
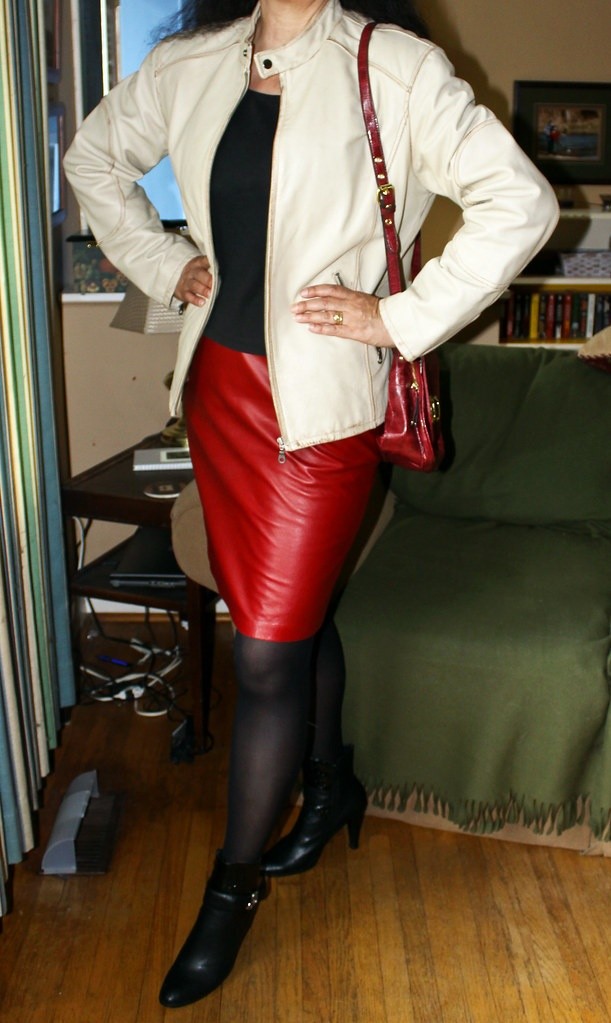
[499,292,611,343]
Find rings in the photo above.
[333,311,343,324]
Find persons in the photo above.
[64,1,559,1008]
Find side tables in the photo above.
[60,430,222,752]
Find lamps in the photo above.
[109,282,188,448]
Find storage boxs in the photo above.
[69,240,131,291]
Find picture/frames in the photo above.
[511,80,611,185]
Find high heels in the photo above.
[266,742,367,876]
[159,850,270,1006]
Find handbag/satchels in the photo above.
[380,347,445,470]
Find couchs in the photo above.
[169,342,610,859]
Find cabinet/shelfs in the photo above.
[493,207,611,351]
